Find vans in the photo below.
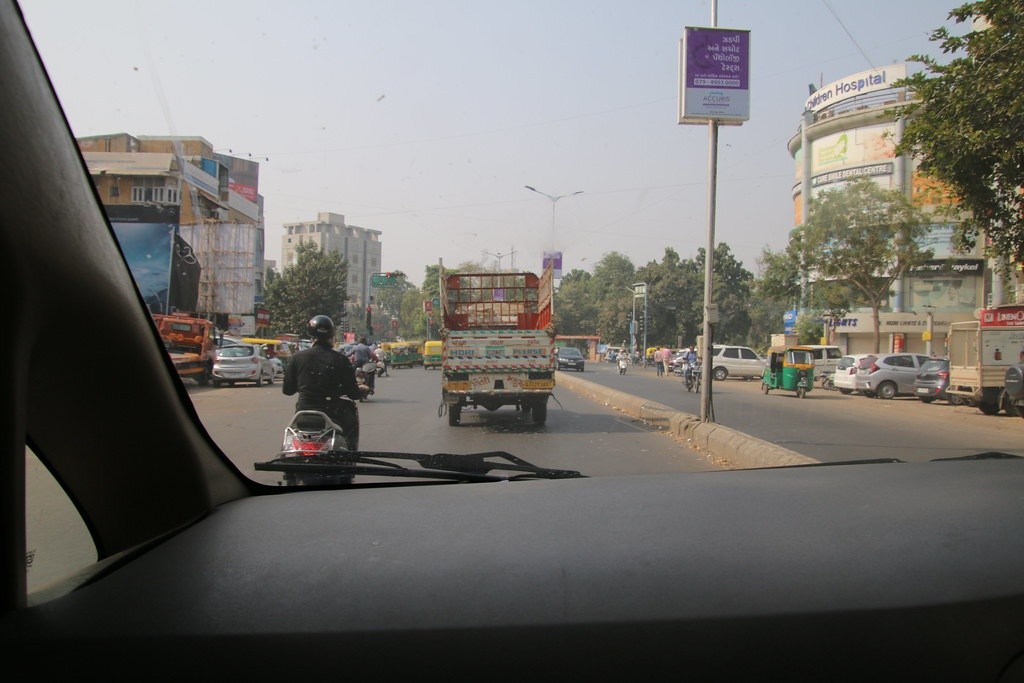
[711,345,766,381]
[801,344,842,382]
[604,347,631,363]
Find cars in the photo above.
[211,343,274,388]
[207,333,312,380]
[668,348,696,373]
[912,357,973,406]
[555,347,586,372]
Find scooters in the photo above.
[376,358,386,377]
[673,361,684,376]
[351,358,374,403]
[820,369,838,391]
[616,357,627,375]
[278,385,374,487]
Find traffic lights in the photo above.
[366,306,371,330]
[385,272,405,278]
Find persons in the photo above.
[682,345,700,386]
[371,344,388,376]
[618,350,624,356]
[345,337,378,396]
[649,352,653,358]
[662,346,671,375]
[283,315,370,476]
[655,347,663,375]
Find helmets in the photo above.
[358,337,365,343]
[377,344,381,348]
[306,315,336,339]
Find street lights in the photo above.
[525,185,584,326]
[485,251,520,288]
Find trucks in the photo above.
[944,321,1024,414]
[437,257,558,427]
[151,311,224,387]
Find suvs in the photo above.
[855,353,932,400]
[833,353,869,396]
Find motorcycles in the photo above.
[646,347,662,367]
[760,345,816,399]
[380,340,442,370]
[682,357,703,393]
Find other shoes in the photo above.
[370,387,374,393]
